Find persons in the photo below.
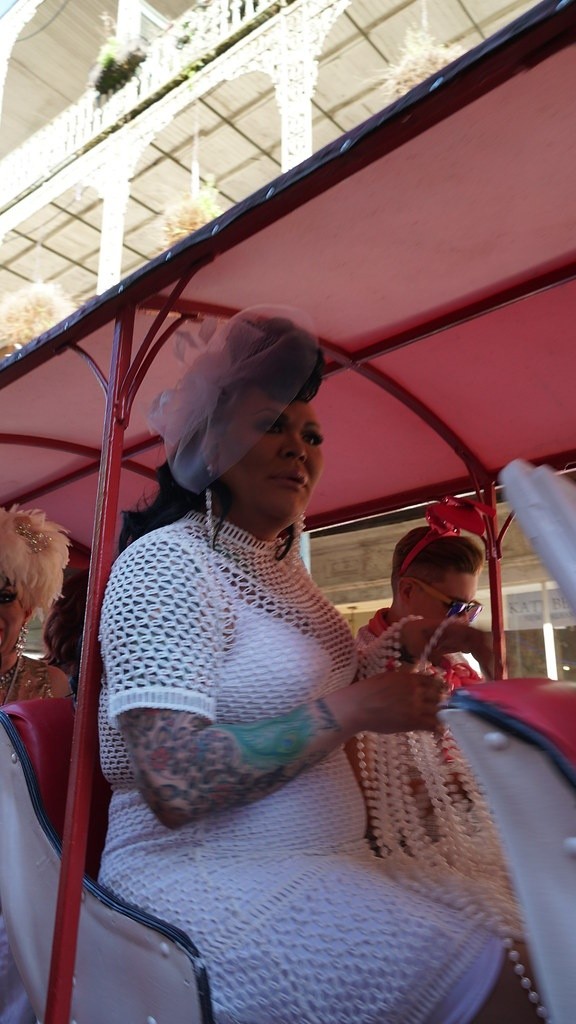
[0,301,542,1022]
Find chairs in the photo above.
[438,676,576,1023]
[0,699,214,1024]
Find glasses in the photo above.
[403,576,484,620]
